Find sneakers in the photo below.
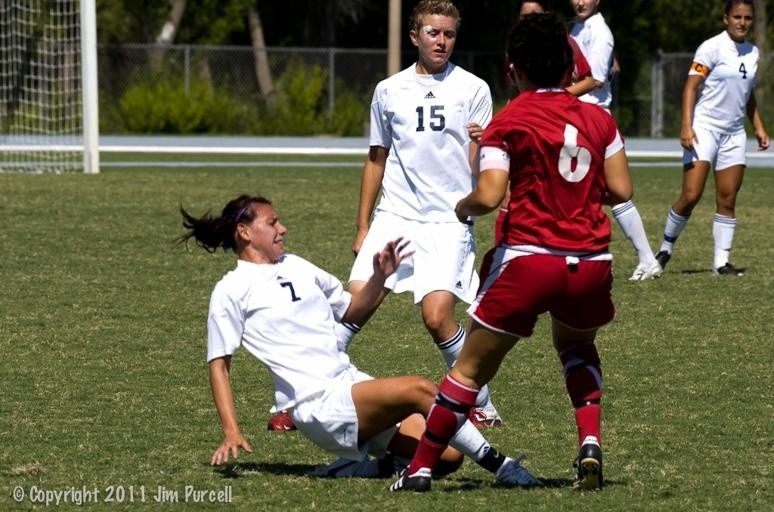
[313,453,368,477]
[628,250,671,281]
[573,435,602,489]
[474,395,502,428]
[496,455,545,487]
[388,464,432,492]
[268,410,294,431]
[712,263,744,276]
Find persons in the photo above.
[561,0,661,281]
[496,2,592,245]
[268,1,508,432]
[393,17,667,489]
[178,195,545,489]
[653,1,772,276]
